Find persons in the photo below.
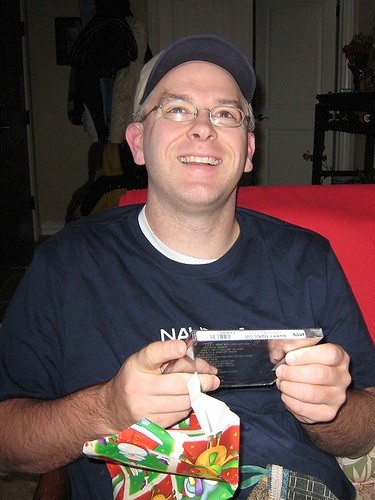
[0,35,374,500]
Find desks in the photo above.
[311,92,375,186]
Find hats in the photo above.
[132,35,257,120]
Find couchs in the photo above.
[0,184,375,500]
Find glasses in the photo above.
[139,99,251,132]
